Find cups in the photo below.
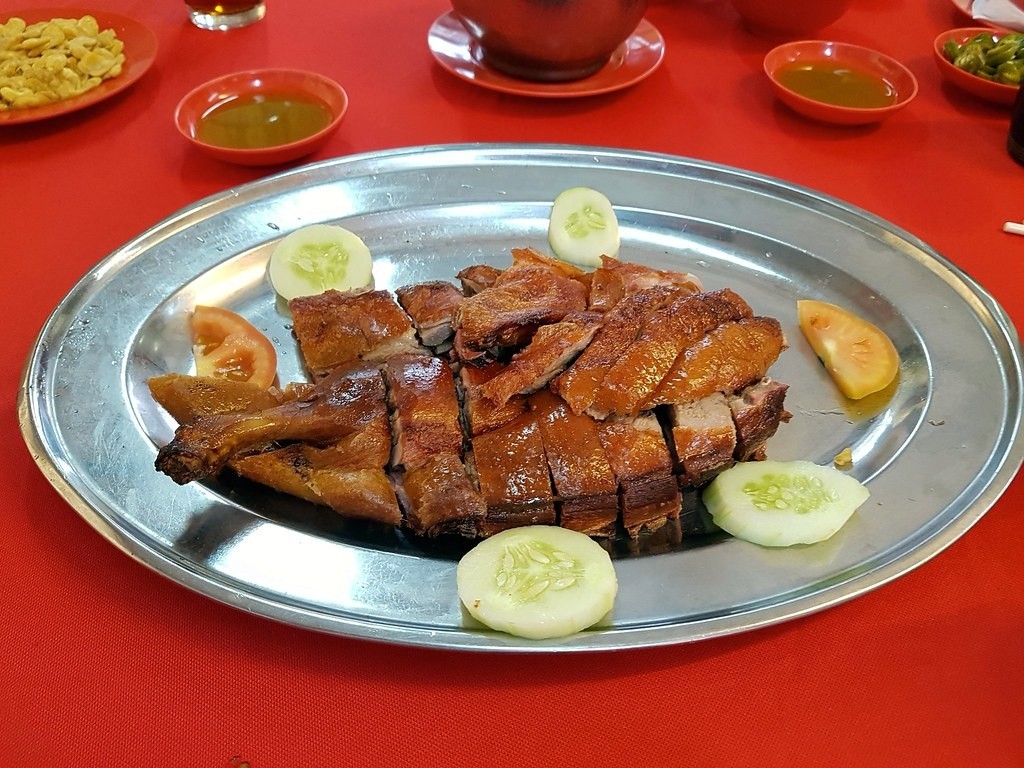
[184,0,267,30]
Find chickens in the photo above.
[145,248,790,536]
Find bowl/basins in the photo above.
[452,0,648,83]
[730,0,852,44]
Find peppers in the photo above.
[944,31,1024,87]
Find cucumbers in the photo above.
[702,460,870,548]
[548,186,620,267]
[457,525,617,640]
[268,224,373,303]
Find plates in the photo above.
[173,68,349,166]
[952,0,1024,34]
[17,142,1024,652]
[427,8,666,99]
[934,27,1024,105]
[763,40,918,125]
[0,0,158,125]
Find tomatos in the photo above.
[796,299,900,399]
[192,305,278,389]
